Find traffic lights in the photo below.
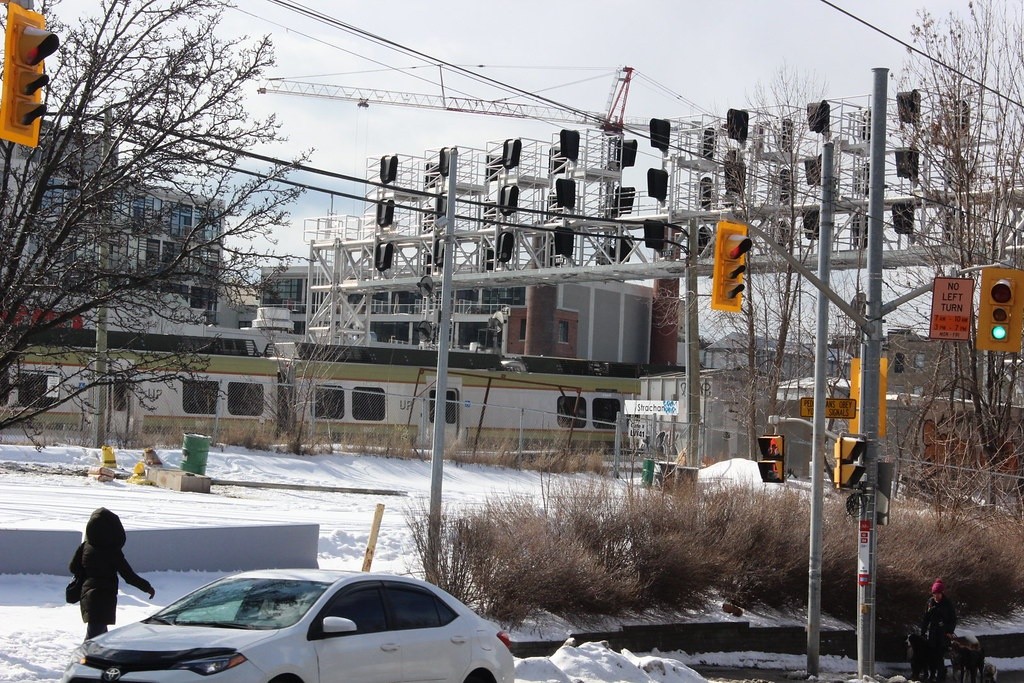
[977,268,1024,352]
[0,2,59,147]
[758,433,786,482]
[711,223,752,312]
[835,436,866,489]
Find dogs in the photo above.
[905,633,985,683]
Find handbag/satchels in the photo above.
[66,580,79,604]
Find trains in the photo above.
[0,326,685,454]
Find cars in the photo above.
[64,569,515,683]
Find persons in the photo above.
[920,579,957,683]
[70,507,155,641]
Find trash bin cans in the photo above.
[675,467,699,496]
[641,458,655,489]
[652,460,677,495]
[181,432,211,476]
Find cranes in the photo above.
[257,0,688,264]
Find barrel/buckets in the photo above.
[674,467,700,497]
[181,432,212,475]
[641,458,657,489]
[651,461,678,493]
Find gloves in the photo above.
[147,588,155,599]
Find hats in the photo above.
[932,579,945,594]
